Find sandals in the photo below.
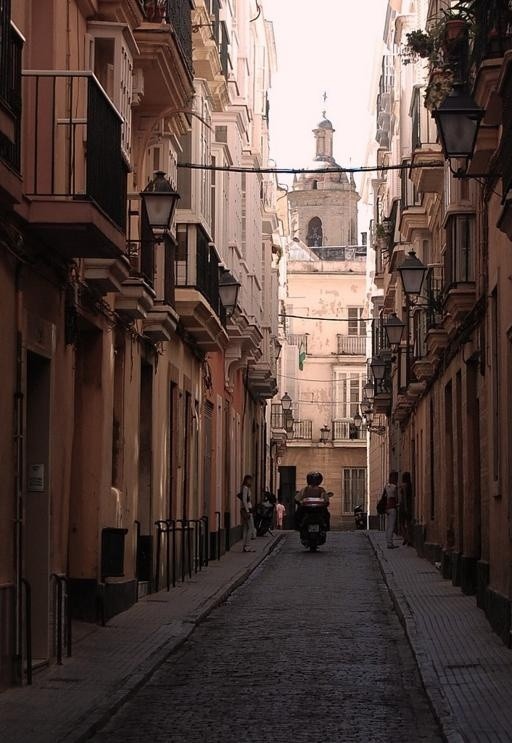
[241,547,256,552]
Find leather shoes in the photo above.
[387,544,400,549]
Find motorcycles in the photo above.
[293,489,334,552]
[253,484,282,537]
[353,502,367,530]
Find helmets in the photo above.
[306,471,323,485]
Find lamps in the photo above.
[281,392,294,432]
[354,411,367,431]
[320,424,331,444]
[360,380,376,418]
[370,355,387,387]
[218,269,241,318]
[381,313,414,354]
[430,81,505,181]
[126,170,181,247]
[396,246,441,309]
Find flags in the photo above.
[298,342,306,371]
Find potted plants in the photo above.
[439,7,468,40]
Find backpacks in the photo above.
[377,488,387,514]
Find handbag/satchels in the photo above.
[248,528,256,539]
[237,492,250,502]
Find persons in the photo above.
[384,472,414,550]
[240,475,258,553]
[274,498,286,530]
[293,471,330,532]
